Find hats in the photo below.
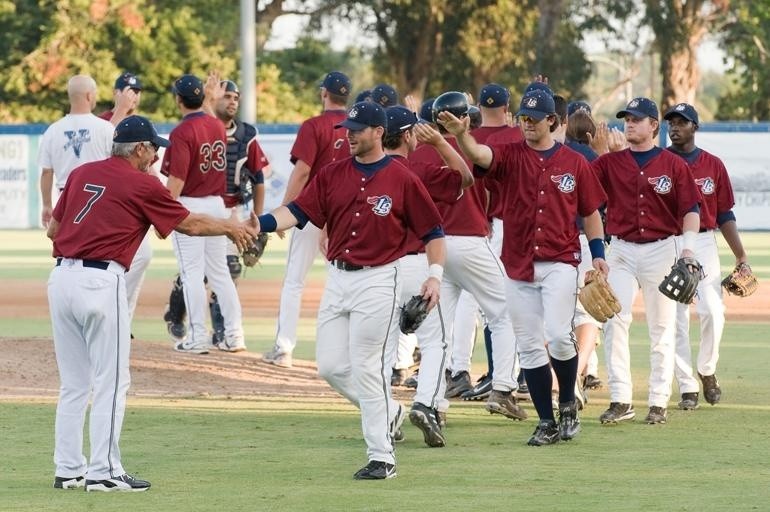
[616,97,659,121]
[567,101,592,117]
[319,72,352,97]
[524,82,555,98]
[171,75,204,98]
[333,101,388,132]
[111,115,171,150]
[480,83,510,108]
[355,90,373,102]
[420,99,436,122]
[384,105,419,135]
[516,90,557,121]
[372,85,398,108]
[664,103,699,125]
[114,74,144,92]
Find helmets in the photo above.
[468,106,482,128]
[431,91,480,123]
[220,80,240,95]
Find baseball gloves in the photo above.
[578,271,622,321]
[658,257,703,305]
[243,232,271,268]
[722,263,758,299]
[399,295,433,335]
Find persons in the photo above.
[39,65,271,353]
[45,114,259,491]
[228,67,748,480]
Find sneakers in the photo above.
[698,370,721,405]
[644,404,671,425]
[487,390,528,422]
[53,469,85,488]
[174,339,210,357]
[677,394,700,410]
[515,382,532,402]
[394,429,406,442]
[408,402,446,448]
[445,374,473,397]
[218,341,246,352]
[460,372,494,401]
[261,349,293,370]
[84,473,152,492]
[164,307,187,341]
[598,402,637,426]
[527,423,559,448]
[212,335,224,346]
[583,374,604,390]
[353,461,398,481]
[391,369,404,386]
[404,370,420,387]
[574,374,588,411]
[557,401,582,440]
[389,402,406,443]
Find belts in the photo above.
[329,258,397,271]
[54,256,112,271]
[616,234,672,244]
[700,228,716,235]
